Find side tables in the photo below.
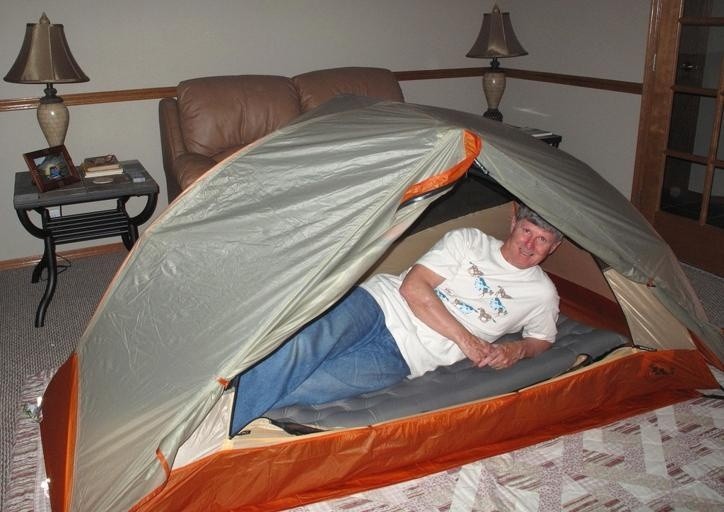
[13,159,160,329]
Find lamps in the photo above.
[465,4,528,122]
[4,11,90,148]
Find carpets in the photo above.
[3,365,724,511]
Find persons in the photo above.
[230,204,564,439]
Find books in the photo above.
[515,126,552,137]
[79,154,145,193]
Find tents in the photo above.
[36,99,724,512]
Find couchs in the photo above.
[157,67,404,205]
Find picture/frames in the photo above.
[22,144,80,193]
